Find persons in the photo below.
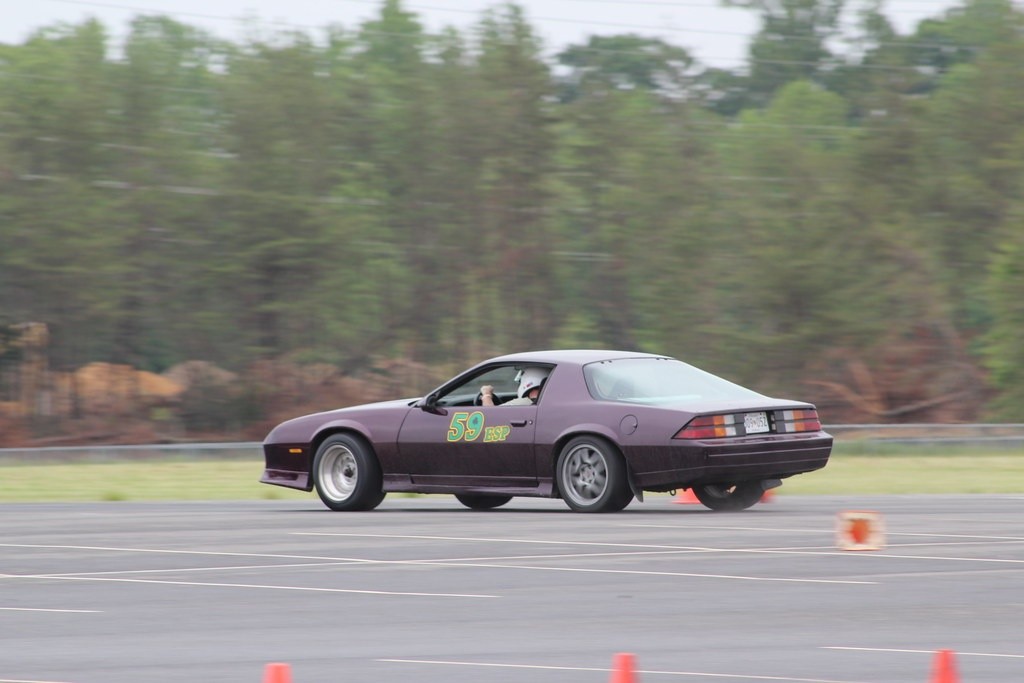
[480,367,551,406]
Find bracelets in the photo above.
[481,394,493,401]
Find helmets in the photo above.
[514,366,550,398]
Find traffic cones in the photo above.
[670,485,701,506]
[929,646,964,683]
[264,661,294,683]
[606,651,640,683]
[759,487,779,503]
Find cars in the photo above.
[257,351,833,514]
